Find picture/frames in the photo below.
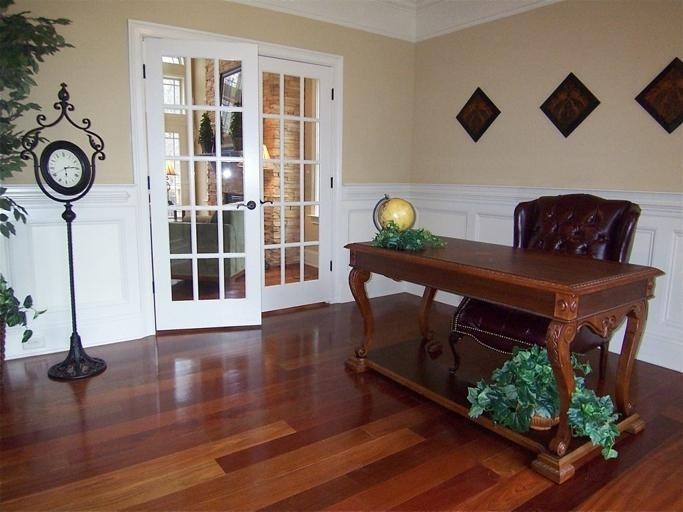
[220,65,242,156]
[456,85,501,143]
[539,71,601,138]
[634,56,683,134]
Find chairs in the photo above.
[448,194,641,386]
[168,200,245,282]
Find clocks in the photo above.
[20,83,107,380]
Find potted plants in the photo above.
[228,101,242,151]
[0,0,75,384]
[198,112,213,153]
[468,343,621,461]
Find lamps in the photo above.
[166,161,180,206]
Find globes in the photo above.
[372,194,416,234]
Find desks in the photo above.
[344,233,665,486]
